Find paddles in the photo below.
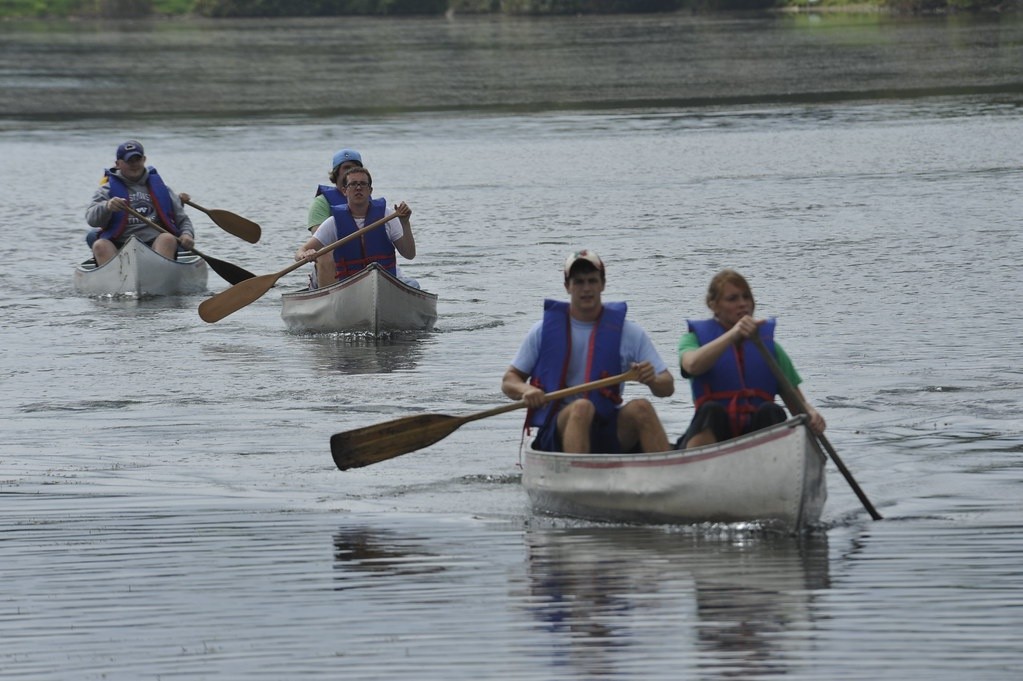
[197,207,406,323]
[126,206,255,287]
[329,366,643,472]
[750,328,883,521]
[182,197,261,243]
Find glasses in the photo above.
[345,182,371,189]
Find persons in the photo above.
[501,249,674,453]
[678,270,826,448]
[295,150,420,292]
[85,140,195,267]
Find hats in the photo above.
[561,249,604,281]
[333,149,363,169]
[116,141,144,162]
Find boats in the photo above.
[280,262,438,335]
[72,234,207,297]
[521,414,829,533]
